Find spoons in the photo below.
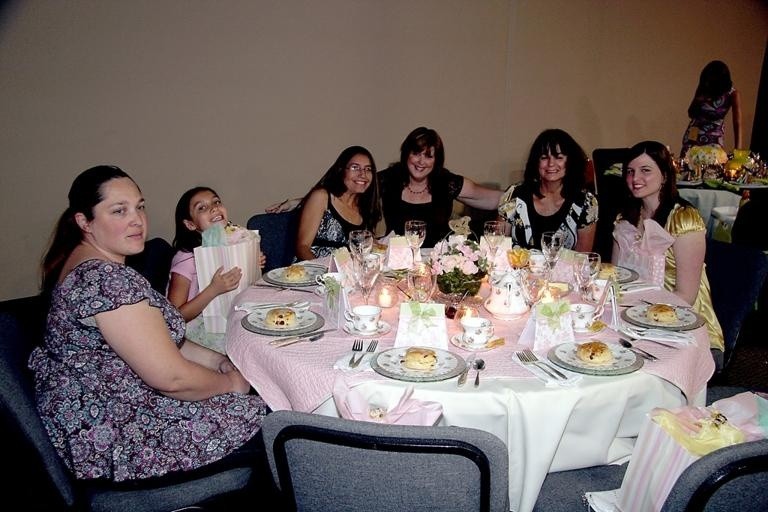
[252,298,311,309]
[473,358,486,387]
[617,338,658,360]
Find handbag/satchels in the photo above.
[613,390,768,512]
[193,229,262,334]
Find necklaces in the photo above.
[404,182,431,195]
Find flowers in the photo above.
[426,235,497,277]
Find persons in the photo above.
[610,141,725,381]
[290,144,382,264]
[162,187,269,355]
[494,129,601,255]
[680,60,743,162]
[30,163,271,490]
[264,125,505,247]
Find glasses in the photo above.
[344,163,371,174]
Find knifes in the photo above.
[251,282,313,293]
[619,305,693,308]
[457,351,477,387]
[269,328,338,345]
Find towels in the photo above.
[332,345,380,372]
[230,299,313,316]
[508,350,584,386]
[614,277,661,294]
[618,322,700,349]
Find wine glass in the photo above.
[349,219,602,317]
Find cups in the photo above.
[314,273,341,288]
[678,158,689,174]
[568,303,604,331]
[460,317,495,345]
[343,306,383,331]
[591,279,614,303]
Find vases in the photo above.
[437,276,482,297]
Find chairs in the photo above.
[125,238,174,296]
[0,295,261,512]
[592,147,768,376]
[247,207,300,276]
[534,438,768,512]
[262,410,510,512]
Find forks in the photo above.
[352,337,378,368]
[515,348,568,381]
[276,333,325,348]
[349,339,364,366]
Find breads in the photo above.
[647,304,675,322]
[405,347,437,369]
[576,341,609,364]
[285,265,306,282]
[265,308,296,328]
[598,264,618,281]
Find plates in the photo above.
[266,265,325,284]
[613,264,632,282]
[375,348,458,376]
[247,310,318,331]
[555,342,636,369]
[626,305,696,327]
[314,286,324,297]
[572,321,607,336]
[450,332,499,351]
[344,321,393,337]
